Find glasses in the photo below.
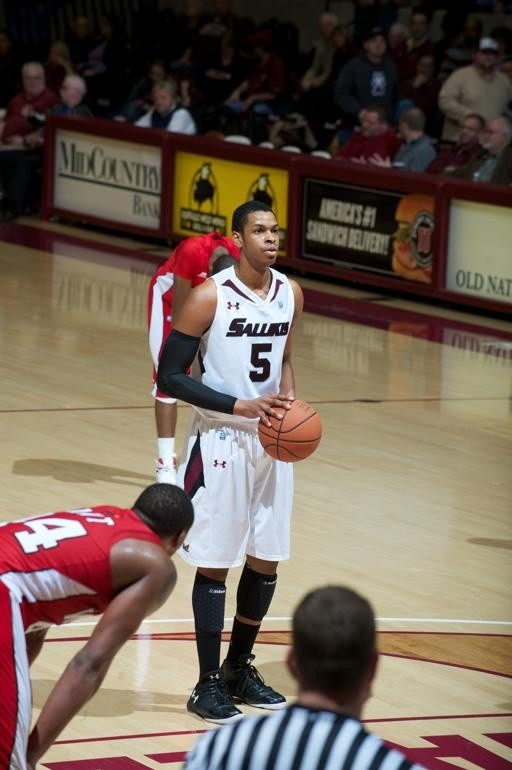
[459,125,481,131]
[482,129,497,134]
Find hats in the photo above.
[475,37,499,54]
[365,25,386,39]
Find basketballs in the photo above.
[257,399,322,462]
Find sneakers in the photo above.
[154,453,177,486]
[187,669,245,724]
[220,654,287,710]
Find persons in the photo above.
[441,113,510,184]
[269,112,317,154]
[184,584,428,770]
[1,478,194,769]
[3,2,291,145]
[437,37,509,152]
[334,26,400,150]
[11,73,93,218]
[333,104,401,166]
[156,199,306,728]
[368,106,437,172]
[296,2,509,138]
[146,231,240,484]
[114,78,198,136]
[425,113,486,179]
[2,61,59,218]
